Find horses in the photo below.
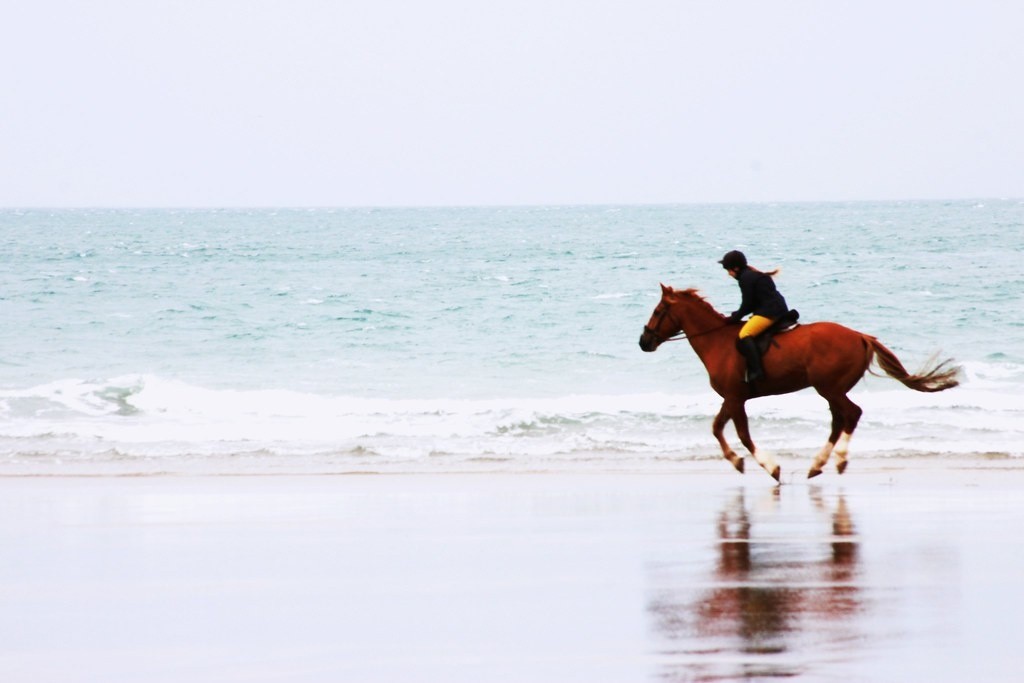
[639,283,961,483]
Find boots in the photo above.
[735,334,767,384]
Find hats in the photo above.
[718,250,747,268]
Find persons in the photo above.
[717,250,788,385]
